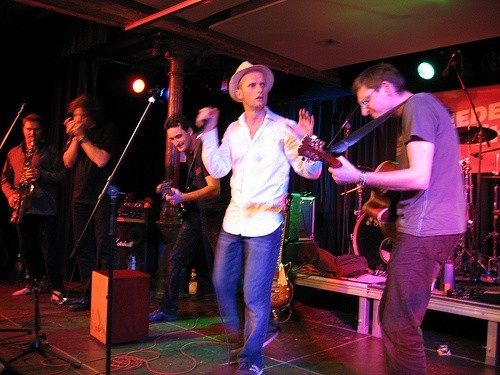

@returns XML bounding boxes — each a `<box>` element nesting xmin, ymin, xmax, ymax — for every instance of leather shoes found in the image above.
<box><xmin>68</xmin><ymin>298</ymin><xmax>90</xmax><ymax>311</ymax></box>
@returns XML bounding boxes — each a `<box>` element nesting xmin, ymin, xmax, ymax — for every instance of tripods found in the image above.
<box><xmin>453</xmin><ymin>136</ymin><xmax>500</xmax><ymax>281</ymax></box>
<box><xmin>4</xmin><ymin>271</ymin><xmax>83</xmax><ymax>370</ymax></box>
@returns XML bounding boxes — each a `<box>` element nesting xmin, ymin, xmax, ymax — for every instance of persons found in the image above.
<box><xmin>1</xmin><ymin>113</ymin><xmax>68</xmax><ymax>295</ymax></box>
<box><xmin>62</xmin><ymin>96</ymin><xmax>115</xmax><ymax>311</ymax></box>
<box><xmin>148</xmin><ymin>110</ymin><xmax>232</xmax><ymax>322</ymax></box>
<box><xmin>328</xmin><ymin>64</ymin><xmax>468</xmax><ymax>375</ymax></box>
<box><xmin>195</xmin><ymin>61</ymin><xmax>322</xmax><ymax>374</ymax></box>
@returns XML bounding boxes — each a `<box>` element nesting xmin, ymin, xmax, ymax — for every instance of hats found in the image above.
<box><xmin>229</xmin><ymin>62</ymin><xmax>274</xmax><ymax>101</ymax></box>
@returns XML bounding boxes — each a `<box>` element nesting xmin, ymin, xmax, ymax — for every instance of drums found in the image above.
<box><xmin>351</xmin><ymin>212</ymin><xmax>391</xmax><ymax>275</ymax></box>
<box><xmin>464</xmin><ymin>202</ymin><xmax>475</xmax><ymax>225</ymax></box>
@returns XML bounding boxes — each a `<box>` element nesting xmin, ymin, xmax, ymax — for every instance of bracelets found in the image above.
<box><xmin>359</xmin><ymin>168</ymin><xmax>368</xmax><ymax>188</ymax></box>
<box><xmin>79</xmin><ymin>136</ymin><xmax>89</xmax><ymax>144</ymax></box>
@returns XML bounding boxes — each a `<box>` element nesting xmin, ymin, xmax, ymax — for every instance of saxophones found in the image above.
<box><xmin>10</xmin><ymin>135</ymin><xmax>36</xmax><ymax>225</ymax></box>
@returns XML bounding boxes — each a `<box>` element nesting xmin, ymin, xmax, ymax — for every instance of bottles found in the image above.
<box><xmin>189</xmin><ymin>268</ymin><xmax>199</xmax><ymax>297</ymax></box>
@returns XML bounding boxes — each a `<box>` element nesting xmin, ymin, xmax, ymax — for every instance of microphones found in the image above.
<box><xmin>443</xmin><ymin>52</ymin><xmax>457</xmax><ymax>77</ymax></box>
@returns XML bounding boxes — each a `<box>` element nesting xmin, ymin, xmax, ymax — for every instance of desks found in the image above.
<box><xmin>116</xmin><ymin>217</ymin><xmax>183</xmax><ymax>226</ymax></box>
<box><xmin>292</xmin><ymin>274</ymin><xmax>500</xmax><ymax>366</ymax></box>
<box><xmin>89</xmin><ymin>269</ymin><xmax>150</xmax><ymax>345</ymax></box>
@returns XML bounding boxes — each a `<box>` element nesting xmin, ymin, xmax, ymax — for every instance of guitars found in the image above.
<box><xmin>294</xmin><ymin>132</ymin><xmax>408</xmax><ymax>242</ymax></box>
<box><xmin>267</xmin><ymin>194</ymin><xmax>294</xmax><ymax>310</ymax></box>
<box><xmin>160</xmin><ymin>179</ymin><xmax>187</xmax><ymax>222</ymax></box>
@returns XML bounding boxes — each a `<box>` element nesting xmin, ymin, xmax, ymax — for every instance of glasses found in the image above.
<box><xmin>359</xmin><ymin>83</ymin><xmax>381</xmax><ymax>108</ymax></box>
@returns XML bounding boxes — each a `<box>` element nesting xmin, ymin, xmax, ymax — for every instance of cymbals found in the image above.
<box><xmin>453</xmin><ymin>126</ymin><xmax>497</xmax><ymax>144</ymax></box>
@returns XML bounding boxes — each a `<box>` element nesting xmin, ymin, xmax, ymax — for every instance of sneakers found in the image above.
<box><xmin>236</xmin><ymin>361</ymin><xmax>262</xmax><ymax>375</ymax></box>
<box><xmin>262</xmin><ymin>329</ymin><xmax>279</xmax><ymax>348</ymax></box>
<box><xmin>148</xmin><ymin>309</ymin><xmax>176</xmax><ymax>322</ymax></box>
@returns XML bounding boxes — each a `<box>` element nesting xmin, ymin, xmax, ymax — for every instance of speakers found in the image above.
<box><xmin>90</xmin><ymin>269</ymin><xmax>151</xmax><ymax>344</ymax></box>
<box><xmin>284</xmin><ymin>192</ymin><xmax>319</xmax><ymax>268</ymax></box>
<box><xmin>114</xmin><ymin>211</ymin><xmax>150</xmax><ymax>272</ymax></box>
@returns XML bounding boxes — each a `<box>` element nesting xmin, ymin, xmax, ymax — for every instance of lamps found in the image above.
<box><xmin>416</xmin><ymin>49</ymin><xmax>443</xmax><ymax>80</ymax></box>
